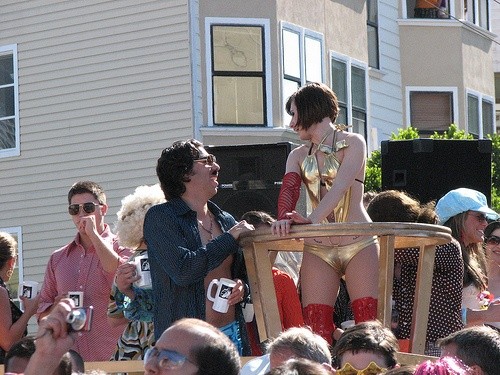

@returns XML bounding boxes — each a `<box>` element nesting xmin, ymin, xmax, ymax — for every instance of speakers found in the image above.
<box><xmin>382</xmin><ymin>138</ymin><xmax>492</xmax><ymax>211</ymax></box>
<box><xmin>203</xmin><ymin>140</ymin><xmax>292</xmax><ymax>223</ymax></box>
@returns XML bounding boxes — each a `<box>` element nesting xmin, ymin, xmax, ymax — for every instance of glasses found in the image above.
<box><xmin>192</xmin><ymin>155</ymin><xmax>216</xmax><ymax>167</ymax></box>
<box><xmin>144</xmin><ymin>346</ymin><xmax>197</xmax><ymax>370</ymax></box>
<box><xmin>335</xmin><ymin>361</ymin><xmax>392</xmax><ymax>375</ymax></box>
<box><xmin>485</xmin><ymin>236</ymin><xmax>500</xmax><ymax>245</ymax></box>
<box><xmin>68</xmin><ymin>202</ymin><xmax>103</xmax><ymax>214</ymax></box>
<box><xmin>467</xmin><ymin>213</ymin><xmax>487</xmax><ymax>222</ymax></box>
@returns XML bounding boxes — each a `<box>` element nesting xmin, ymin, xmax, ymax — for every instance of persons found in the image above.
<box><xmin>0</xmin><ymin>81</ymin><xmax>500</xmax><ymax>375</ymax></box>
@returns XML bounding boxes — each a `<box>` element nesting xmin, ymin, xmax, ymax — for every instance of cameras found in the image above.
<box><xmin>65</xmin><ymin>306</ymin><xmax>94</xmax><ymax>334</ymax></box>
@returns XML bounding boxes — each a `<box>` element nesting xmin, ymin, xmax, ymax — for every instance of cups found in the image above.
<box><xmin>21</xmin><ymin>281</ymin><xmax>37</xmax><ymax>309</ymax></box>
<box><xmin>68</xmin><ymin>291</ymin><xmax>83</xmax><ymax>308</ymax></box>
<box><xmin>207</xmin><ymin>277</ymin><xmax>237</xmax><ymax>313</ymax></box>
<box><xmin>130</xmin><ymin>256</ymin><xmax>153</xmax><ymax>289</ymax></box>
<box><xmin>341</xmin><ymin>320</ymin><xmax>356</xmax><ymax>332</ymax></box>
<box><xmin>241</xmin><ymin>295</ymin><xmax>254</xmax><ymax>323</ymax></box>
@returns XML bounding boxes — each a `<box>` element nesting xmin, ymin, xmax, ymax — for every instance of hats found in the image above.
<box><xmin>433</xmin><ymin>188</ymin><xmax>499</xmax><ymax>225</ymax></box>
